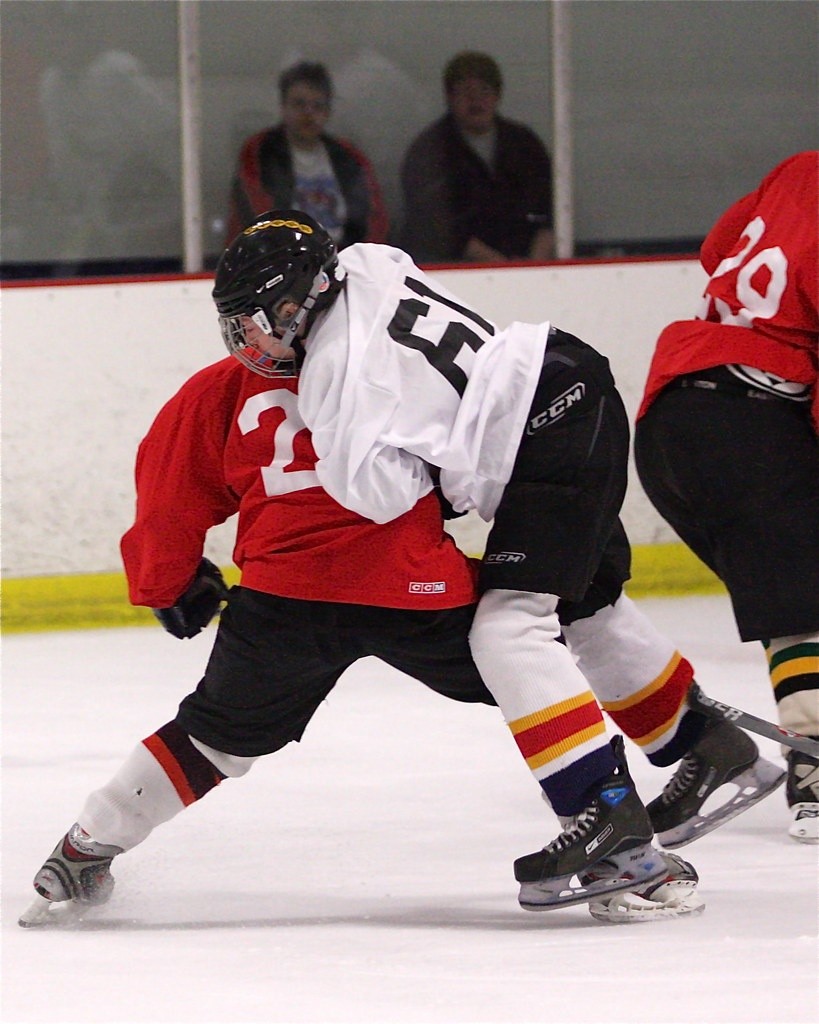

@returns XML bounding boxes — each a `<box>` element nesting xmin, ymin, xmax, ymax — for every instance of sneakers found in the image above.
<box><xmin>646</xmin><ymin>680</ymin><xmax>788</xmax><ymax>849</ymax></box>
<box><xmin>512</xmin><ymin>735</ymin><xmax>669</xmax><ymax>913</ymax></box>
<box><xmin>785</xmin><ymin>734</ymin><xmax>819</xmax><ymax>839</ymax></box>
<box><xmin>582</xmin><ymin>849</ymin><xmax>707</xmax><ymax>923</ymax></box>
<box><xmin>18</xmin><ymin>822</ymin><xmax>126</xmax><ymax>926</ymax></box>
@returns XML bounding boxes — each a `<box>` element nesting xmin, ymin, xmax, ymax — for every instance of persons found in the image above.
<box><xmin>221</xmin><ymin>59</ymin><xmax>389</xmax><ymax>254</ymax></box>
<box><xmin>212</xmin><ymin>208</ymin><xmax>786</xmax><ymax>884</ymax></box>
<box><xmin>11</xmin><ymin>337</ymin><xmax>698</xmax><ymax>903</ymax></box>
<box><xmin>397</xmin><ymin>51</ymin><xmax>555</xmax><ymax>269</ymax></box>
<box><xmin>635</xmin><ymin>148</ymin><xmax>819</xmax><ymax>845</ymax></box>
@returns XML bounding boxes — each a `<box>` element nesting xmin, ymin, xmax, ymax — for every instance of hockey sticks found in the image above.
<box><xmin>556</xmin><ymin>631</ymin><xmax>819</xmax><ymax>760</ymax></box>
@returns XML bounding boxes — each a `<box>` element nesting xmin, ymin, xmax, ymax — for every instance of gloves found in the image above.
<box><xmin>424</xmin><ymin>464</ymin><xmax>471</xmax><ymax>520</ymax></box>
<box><xmin>153</xmin><ymin>558</ymin><xmax>225</xmax><ymax>639</ymax></box>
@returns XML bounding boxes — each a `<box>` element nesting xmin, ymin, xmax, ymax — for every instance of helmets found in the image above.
<box><xmin>212</xmin><ymin>209</ymin><xmax>349</xmax><ymax>379</ymax></box>
